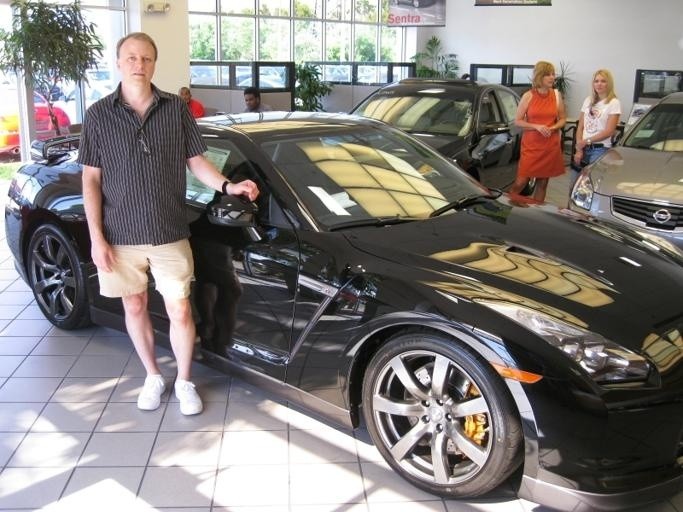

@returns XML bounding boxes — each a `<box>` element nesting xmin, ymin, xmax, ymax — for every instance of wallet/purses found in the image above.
<box><xmin>571</xmin><ymin>159</ymin><xmax>589</xmax><ymax>173</ymax></box>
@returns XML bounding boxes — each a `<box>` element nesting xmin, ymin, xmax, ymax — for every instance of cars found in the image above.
<box><xmin>4</xmin><ymin>110</ymin><xmax>683</xmax><ymax>511</ymax></box>
<box><xmin>347</xmin><ymin>79</ymin><xmax>538</xmax><ymax>197</ymax></box>
<box><xmin>212</xmin><ymin>66</ymin><xmax>282</xmax><ymax>85</ymax></box>
<box><xmin>63</xmin><ymin>69</ymin><xmax>111</xmax><ymax>100</ymax></box>
<box><xmin>192</xmin><ymin>66</ymin><xmax>216</xmax><ymax>84</ymax></box>
<box><xmin>568</xmin><ymin>92</ymin><xmax>683</xmax><ymax>251</ymax></box>
<box><xmin>53</xmin><ymin>80</ymin><xmax>114</xmax><ymax>124</ymax></box>
<box><xmin>0</xmin><ymin>83</ymin><xmax>76</xmax><ymax>154</ymax></box>
<box><xmin>33</xmin><ymin>79</ymin><xmax>60</xmax><ymax>101</ymax></box>
<box><xmin>327</xmin><ymin>66</ymin><xmax>363</xmax><ymax>81</ymax></box>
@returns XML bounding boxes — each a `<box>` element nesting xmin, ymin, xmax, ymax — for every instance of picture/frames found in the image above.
<box><xmin>473</xmin><ymin>0</ymin><xmax>552</xmax><ymax>7</ymax></box>
<box><xmin>385</xmin><ymin>0</ymin><xmax>445</xmax><ymax>28</ymax></box>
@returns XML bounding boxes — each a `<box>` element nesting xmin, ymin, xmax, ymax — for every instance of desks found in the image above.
<box><xmin>559</xmin><ymin>118</ymin><xmax>626</xmax><ymax>166</ymax></box>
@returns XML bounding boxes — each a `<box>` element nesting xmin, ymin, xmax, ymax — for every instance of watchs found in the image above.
<box><xmin>222</xmin><ymin>178</ymin><xmax>231</xmax><ymax>197</ymax></box>
<box><xmin>587</xmin><ymin>137</ymin><xmax>591</xmax><ymax>145</ymax></box>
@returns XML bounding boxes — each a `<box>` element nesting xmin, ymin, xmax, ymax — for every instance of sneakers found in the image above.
<box><xmin>175</xmin><ymin>379</ymin><xmax>203</xmax><ymax>415</ymax></box>
<box><xmin>138</xmin><ymin>374</ymin><xmax>166</xmax><ymax>411</ymax></box>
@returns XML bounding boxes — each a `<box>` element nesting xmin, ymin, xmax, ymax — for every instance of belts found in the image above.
<box><xmin>586</xmin><ymin>143</ymin><xmax>603</xmax><ymax>148</ymax></box>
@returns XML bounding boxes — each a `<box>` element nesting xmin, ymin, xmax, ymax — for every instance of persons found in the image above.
<box><xmin>510</xmin><ymin>62</ymin><xmax>567</xmax><ymax>204</ymax></box>
<box><xmin>179</xmin><ymin>87</ymin><xmax>204</xmax><ymax>119</ymax></box>
<box><xmin>244</xmin><ymin>86</ymin><xmax>272</xmax><ymax>113</ymax></box>
<box><xmin>573</xmin><ymin>69</ymin><xmax>622</xmax><ymax>167</ymax></box>
<box><xmin>77</xmin><ymin>31</ymin><xmax>260</xmax><ymax>416</ymax></box>
